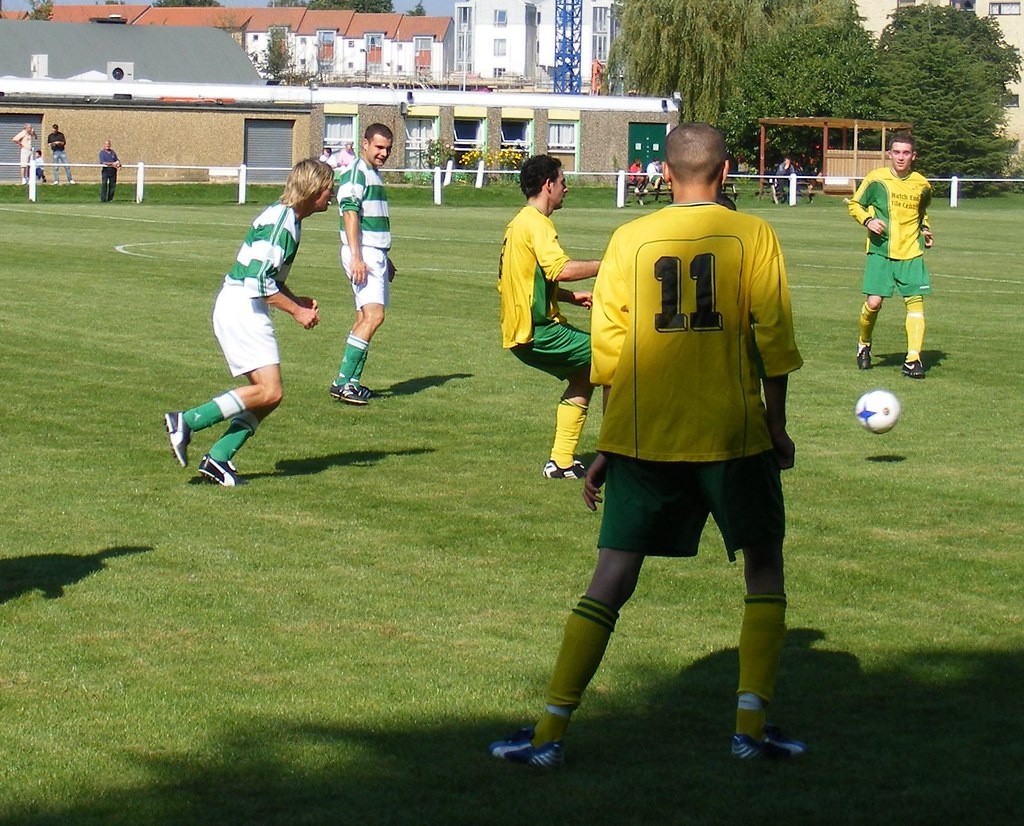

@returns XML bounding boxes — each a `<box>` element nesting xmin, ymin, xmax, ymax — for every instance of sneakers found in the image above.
<box><xmin>542</xmin><ymin>460</ymin><xmax>588</xmax><ymax>479</ymax></box>
<box><xmin>857</xmin><ymin>343</ymin><xmax>871</xmax><ymax>369</ymax></box>
<box><xmin>356</xmin><ymin>383</ymin><xmax>376</xmax><ymax>399</ymax></box>
<box><xmin>164</xmin><ymin>411</ymin><xmax>192</xmax><ymax>468</ymax></box>
<box><xmin>901</xmin><ymin>359</ymin><xmax>926</xmax><ymax>378</ymax></box>
<box><xmin>732</xmin><ymin>725</ymin><xmax>809</xmax><ymax>759</ymax></box>
<box><xmin>330</xmin><ymin>380</ymin><xmax>369</xmax><ymax>404</ymax></box>
<box><xmin>488</xmin><ymin>728</ymin><xmax>566</xmax><ymax>771</ymax></box>
<box><xmin>197</xmin><ymin>454</ymin><xmax>247</xmax><ymax>486</ymax></box>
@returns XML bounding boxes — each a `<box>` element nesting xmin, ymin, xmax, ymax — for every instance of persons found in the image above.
<box><xmin>98</xmin><ymin>140</ymin><xmax>121</xmax><ymax>202</ymax></box>
<box><xmin>35</xmin><ymin>150</ymin><xmax>47</xmax><ymax>183</ymax></box>
<box><xmin>498</xmin><ymin>155</ymin><xmax>602</xmax><ymax>481</ymax></box>
<box><xmin>338</xmin><ymin>140</ymin><xmax>356</xmax><ymax>168</ymax></box>
<box><xmin>330</xmin><ymin>123</ymin><xmax>398</xmax><ymax>405</ymax></box>
<box><xmin>647</xmin><ymin>158</ymin><xmax>663</xmax><ymax>189</ymax></box>
<box><xmin>166</xmin><ymin>160</ymin><xmax>335</xmax><ymax>487</ymax></box>
<box><xmin>488</xmin><ymin>122</ymin><xmax>808</xmax><ymax>769</ymax></box>
<box><xmin>848</xmin><ymin>136</ymin><xmax>934</xmax><ymax>378</ymax></box>
<box><xmin>630</xmin><ymin>158</ymin><xmax>649</xmax><ymax>193</ymax></box>
<box><xmin>47</xmin><ymin>124</ymin><xmax>76</xmax><ymax>185</ymax></box>
<box><xmin>320</xmin><ymin>148</ymin><xmax>337</xmax><ymax>168</ymax></box>
<box><xmin>775</xmin><ymin>156</ymin><xmax>794</xmax><ymax>191</ymax></box>
<box><xmin>13</xmin><ymin>123</ymin><xmax>38</xmax><ymax>185</ymax></box>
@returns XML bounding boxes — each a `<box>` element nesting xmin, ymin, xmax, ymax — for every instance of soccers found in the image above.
<box><xmin>853</xmin><ymin>390</ymin><xmax>902</xmax><ymax>434</ymax></box>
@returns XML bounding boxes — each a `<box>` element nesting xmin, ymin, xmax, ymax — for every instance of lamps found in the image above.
<box><xmin>400</xmin><ymin>102</ymin><xmax>407</xmax><ymax>121</ymax></box>
<box><xmin>310</xmin><ymin>79</ymin><xmax>319</xmax><ymax>105</ymax></box>
<box><xmin>662</xmin><ymin>100</ymin><xmax>667</xmax><ymax>108</ymax></box>
<box><xmin>407</xmin><ymin>92</ymin><xmax>412</xmax><ymax>100</ymax></box>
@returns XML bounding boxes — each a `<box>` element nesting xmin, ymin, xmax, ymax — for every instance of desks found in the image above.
<box><xmin>623</xmin><ymin>182</ymin><xmax>739</xmax><ymax>203</ymax></box>
<box><xmin>758</xmin><ymin>181</ymin><xmax>817</xmax><ymax>203</ymax></box>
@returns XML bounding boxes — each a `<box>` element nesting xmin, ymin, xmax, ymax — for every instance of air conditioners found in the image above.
<box><xmin>107</xmin><ymin>61</ymin><xmax>136</xmax><ymax>82</ymax></box>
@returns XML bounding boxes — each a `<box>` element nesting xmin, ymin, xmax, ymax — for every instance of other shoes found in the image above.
<box><xmin>68</xmin><ymin>179</ymin><xmax>76</xmax><ymax>184</ymax></box>
<box><xmin>54</xmin><ymin>181</ymin><xmax>59</xmax><ymax>185</ymax></box>
<box><xmin>634</xmin><ymin>188</ymin><xmax>640</xmax><ymax>193</ymax></box>
<box><xmin>642</xmin><ymin>190</ymin><xmax>649</xmax><ymax>194</ymax></box>
<box><xmin>22</xmin><ymin>178</ymin><xmax>28</xmax><ymax>184</ymax></box>
<box><xmin>655</xmin><ymin>189</ymin><xmax>661</xmax><ymax>193</ymax></box>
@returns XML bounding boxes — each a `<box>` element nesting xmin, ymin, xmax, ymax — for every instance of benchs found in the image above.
<box><xmin>624</xmin><ymin>189</ymin><xmax>820</xmax><ymax>198</ymax></box>
